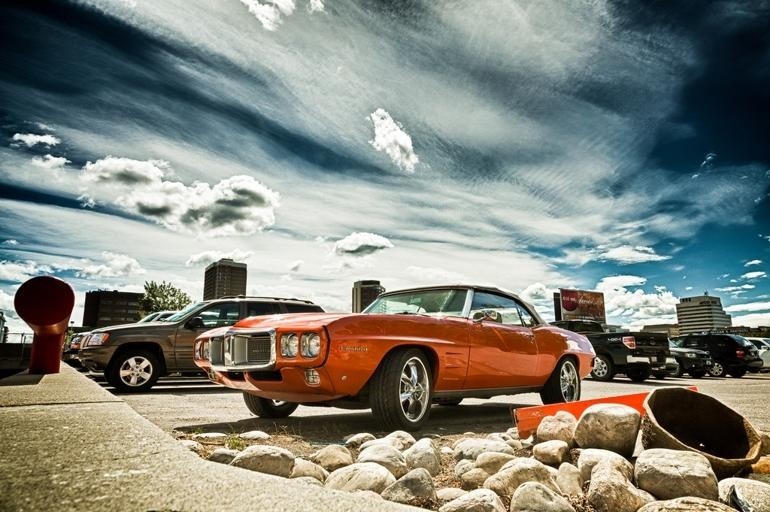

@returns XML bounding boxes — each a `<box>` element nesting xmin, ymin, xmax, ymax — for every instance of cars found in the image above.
<box><xmin>664</xmin><ymin>333</ymin><xmax>770</xmax><ymax>377</ymax></box>
<box><xmin>60</xmin><ymin>311</ymin><xmax>240</xmax><ymax>388</ymax></box>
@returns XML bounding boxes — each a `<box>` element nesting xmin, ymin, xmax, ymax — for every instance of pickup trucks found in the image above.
<box><xmin>544</xmin><ymin>320</ymin><xmax>671</xmax><ymax>381</ymax></box>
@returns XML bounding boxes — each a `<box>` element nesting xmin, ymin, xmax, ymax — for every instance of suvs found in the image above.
<box><xmin>78</xmin><ymin>294</ymin><xmax>326</xmax><ymax>393</ymax></box>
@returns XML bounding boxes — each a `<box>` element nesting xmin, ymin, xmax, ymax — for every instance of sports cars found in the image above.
<box><xmin>192</xmin><ymin>286</ymin><xmax>598</xmax><ymax>432</ymax></box>
